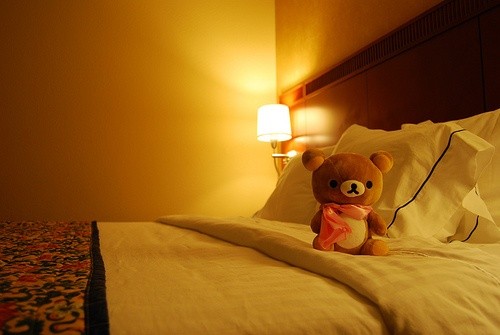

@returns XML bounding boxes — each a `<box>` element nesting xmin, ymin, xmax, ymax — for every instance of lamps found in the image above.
<box><xmin>257</xmin><ymin>104</ymin><xmax>293</xmax><ymax>177</ymax></box>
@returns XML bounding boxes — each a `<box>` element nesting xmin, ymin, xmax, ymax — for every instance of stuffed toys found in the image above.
<box><xmin>301</xmin><ymin>150</ymin><xmax>394</xmax><ymax>256</ymax></box>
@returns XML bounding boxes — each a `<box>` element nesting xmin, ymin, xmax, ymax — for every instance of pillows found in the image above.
<box><xmin>252</xmin><ymin>144</ymin><xmax>336</xmax><ymax>226</ymax></box>
<box><xmin>330</xmin><ymin>123</ymin><xmax>498</xmax><ymax>243</ymax></box>
<box><xmin>401</xmin><ymin>108</ymin><xmax>500</xmax><ymax>244</ymax></box>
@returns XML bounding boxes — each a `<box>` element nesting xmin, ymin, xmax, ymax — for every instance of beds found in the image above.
<box><xmin>0</xmin><ymin>214</ymin><xmax>500</xmax><ymax>335</ymax></box>
